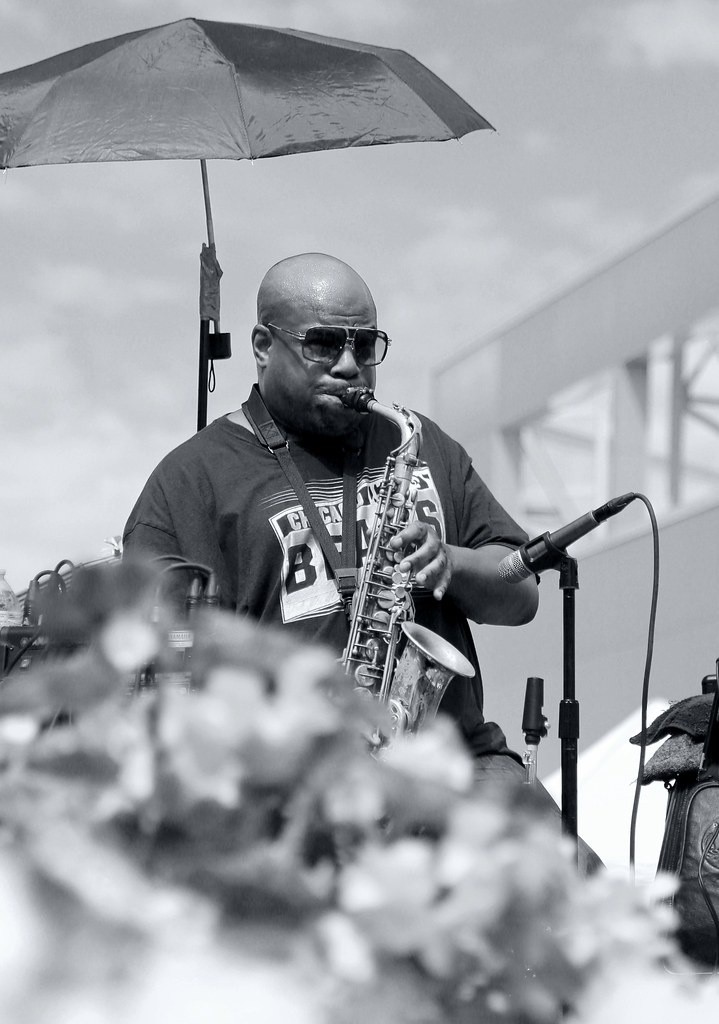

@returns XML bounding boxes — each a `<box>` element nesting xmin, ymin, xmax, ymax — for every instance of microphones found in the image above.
<box><xmin>497</xmin><ymin>493</ymin><xmax>635</xmax><ymax>585</ymax></box>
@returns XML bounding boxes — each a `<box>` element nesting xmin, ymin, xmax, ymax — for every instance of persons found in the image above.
<box><xmin>121</xmin><ymin>252</ymin><xmax>609</xmax><ymax>869</ymax></box>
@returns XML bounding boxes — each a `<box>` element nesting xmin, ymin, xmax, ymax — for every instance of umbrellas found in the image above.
<box><xmin>0</xmin><ymin>18</ymin><xmax>496</xmax><ymax>432</ymax></box>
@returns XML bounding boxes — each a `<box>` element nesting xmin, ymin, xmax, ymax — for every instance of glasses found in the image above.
<box><xmin>261</xmin><ymin>320</ymin><xmax>390</xmax><ymax>367</ymax></box>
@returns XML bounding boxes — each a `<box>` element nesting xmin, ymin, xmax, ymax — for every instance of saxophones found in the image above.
<box><xmin>337</xmin><ymin>384</ymin><xmax>479</xmax><ymax>760</ymax></box>
